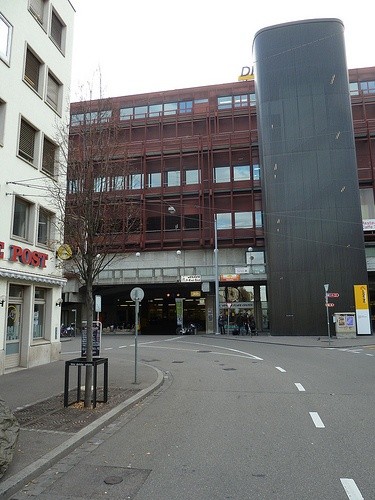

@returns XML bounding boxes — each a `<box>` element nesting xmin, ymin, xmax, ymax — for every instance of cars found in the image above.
<box><xmin>175</xmin><ymin>324</ymin><xmax>196</xmax><ymax>335</ymax></box>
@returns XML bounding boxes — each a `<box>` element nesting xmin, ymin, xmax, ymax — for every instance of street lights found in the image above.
<box><xmin>72</xmin><ymin>309</ymin><xmax>76</xmax><ymax>337</ymax></box>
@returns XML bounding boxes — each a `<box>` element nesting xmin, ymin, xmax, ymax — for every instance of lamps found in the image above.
<box><xmin>248</xmin><ymin>247</ymin><xmax>254</xmax><ymax>273</ymax></box>
<box><xmin>56</xmin><ymin>298</ymin><xmax>64</xmax><ymax>307</ymax></box>
<box><xmin>96</xmin><ymin>253</ymin><xmax>102</xmax><ymax>268</ymax></box>
<box><xmin>135</xmin><ymin>252</ymin><xmax>141</xmax><ymax>271</ymax></box>
<box><xmin>176</xmin><ymin>250</ymin><xmax>181</xmax><ymax>269</ymax></box>
<box><xmin>168</xmin><ymin>205</ymin><xmax>175</xmax><ymax>212</ymax></box>
<box><xmin>0</xmin><ymin>294</ymin><xmax>6</xmax><ymax>307</ymax></box>
